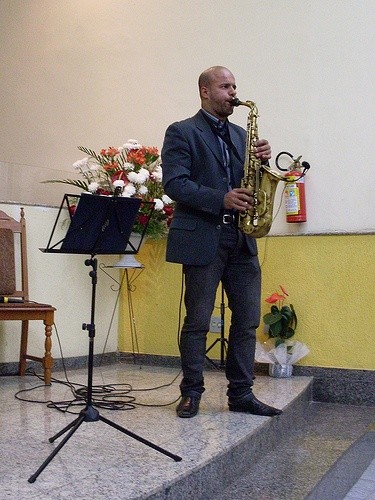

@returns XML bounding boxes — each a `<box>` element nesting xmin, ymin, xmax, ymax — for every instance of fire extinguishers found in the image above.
<box><xmin>275</xmin><ymin>150</ymin><xmax>311</xmax><ymax>222</ymax></box>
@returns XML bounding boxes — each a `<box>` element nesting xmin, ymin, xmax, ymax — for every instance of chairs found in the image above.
<box><xmin>0</xmin><ymin>206</ymin><xmax>58</xmax><ymax>386</ymax></box>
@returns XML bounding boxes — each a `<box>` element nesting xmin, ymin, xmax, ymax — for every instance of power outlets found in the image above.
<box><xmin>208</xmin><ymin>315</ymin><xmax>227</xmax><ymax>333</ymax></box>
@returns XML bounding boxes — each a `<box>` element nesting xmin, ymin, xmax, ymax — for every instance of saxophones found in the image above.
<box><xmin>230</xmin><ymin>96</ymin><xmax>284</xmax><ymax>238</ymax></box>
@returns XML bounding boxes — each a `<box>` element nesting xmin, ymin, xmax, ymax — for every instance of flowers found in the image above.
<box><xmin>254</xmin><ymin>285</ymin><xmax>310</xmax><ymax>366</ymax></box>
<box><xmin>38</xmin><ymin>139</ymin><xmax>175</xmax><ymax>242</ymax></box>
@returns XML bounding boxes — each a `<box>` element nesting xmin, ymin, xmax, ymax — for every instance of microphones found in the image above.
<box><xmin>291</xmin><ymin>161</ymin><xmax>310</xmax><ymax>181</ymax></box>
<box><xmin>0</xmin><ymin>296</ymin><xmax>25</xmax><ymax>303</ymax></box>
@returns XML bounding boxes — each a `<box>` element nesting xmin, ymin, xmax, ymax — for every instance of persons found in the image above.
<box><xmin>160</xmin><ymin>65</ymin><xmax>283</xmax><ymax>418</ymax></box>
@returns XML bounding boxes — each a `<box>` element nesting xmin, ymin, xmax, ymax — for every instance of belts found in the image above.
<box><xmin>222</xmin><ymin>214</ymin><xmax>236</xmax><ymax>224</ymax></box>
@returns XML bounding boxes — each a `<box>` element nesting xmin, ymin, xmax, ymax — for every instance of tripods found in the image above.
<box><xmin>204</xmin><ymin>282</ymin><xmax>256</xmax><ymax>381</ymax></box>
<box><xmin>27</xmin><ymin>192</ymin><xmax>181</xmax><ymax>484</ymax></box>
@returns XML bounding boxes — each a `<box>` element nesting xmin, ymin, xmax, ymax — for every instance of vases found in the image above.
<box><xmin>268</xmin><ymin>362</ymin><xmax>293</xmax><ymax>378</ymax></box>
<box><xmin>112</xmin><ymin>231</ymin><xmax>147</xmax><ymax>269</ymax></box>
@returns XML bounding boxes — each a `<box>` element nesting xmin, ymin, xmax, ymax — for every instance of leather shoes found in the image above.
<box><xmin>176</xmin><ymin>399</ymin><xmax>199</xmax><ymax>418</ymax></box>
<box><xmin>228</xmin><ymin>394</ymin><xmax>283</xmax><ymax>416</ymax></box>
